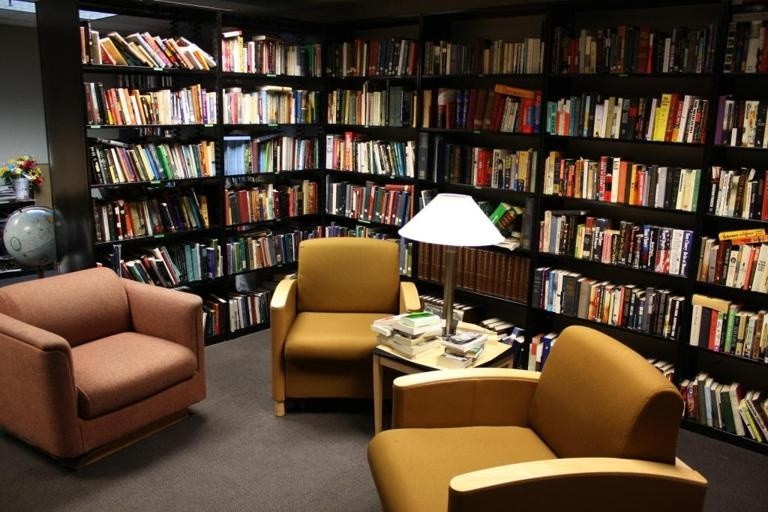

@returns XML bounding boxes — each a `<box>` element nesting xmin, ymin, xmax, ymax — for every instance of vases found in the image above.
<box><xmin>12</xmin><ymin>176</ymin><xmax>30</xmax><ymax>200</ymax></box>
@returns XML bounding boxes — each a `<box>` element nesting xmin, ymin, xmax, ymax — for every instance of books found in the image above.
<box><xmin>79</xmin><ymin>11</ymin><xmax>767</xmax><ymax>445</ymax></box>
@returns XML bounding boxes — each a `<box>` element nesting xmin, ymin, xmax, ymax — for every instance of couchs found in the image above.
<box><xmin>3</xmin><ymin>266</ymin><xmax>208</xmax><ymax>470</ymax></box>
<box><xmin>269</xmin><ymin>237</ymin><xmax>421</xmax><ymax>418</ymax></box>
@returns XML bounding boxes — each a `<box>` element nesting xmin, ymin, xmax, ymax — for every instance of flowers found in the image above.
<box><xmin>0</xmin><ymin>150</ymin><xmax>45</xmax><ymax>186</ymax></box>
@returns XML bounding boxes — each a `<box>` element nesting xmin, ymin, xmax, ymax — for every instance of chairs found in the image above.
<box><xmin>367</xmin><ymin>321</ymin><xmax>709</xmax><ymax>510</ymax></box>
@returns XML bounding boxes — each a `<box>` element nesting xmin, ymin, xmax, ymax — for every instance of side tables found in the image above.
<box><xmin>370</xmin><ymin>322</ymin><xmax>514</xmax><ymax>444</ymax></box>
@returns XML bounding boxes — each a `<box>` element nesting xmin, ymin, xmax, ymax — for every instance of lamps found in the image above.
<box><xmin>395</xmin><ymin>195</ymin><xmax>512</xmax><ymax>346</ymax></box>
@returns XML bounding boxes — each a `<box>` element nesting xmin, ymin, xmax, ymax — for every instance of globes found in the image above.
<box><xmin>2</xmin><ymin>205</ymin><xmax>57</xmax><ymax>278</ymax></box>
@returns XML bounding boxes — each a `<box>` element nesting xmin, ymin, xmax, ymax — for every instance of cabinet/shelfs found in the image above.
<box><xmin>319</xmin><ymin>2</ymin><xmax>766</xmax><ymax>454</ymax></box>
<box><xmin>35</xmin><ymin>2</ymin><xmax>325</xmax><ymax>347</ymax></box>
